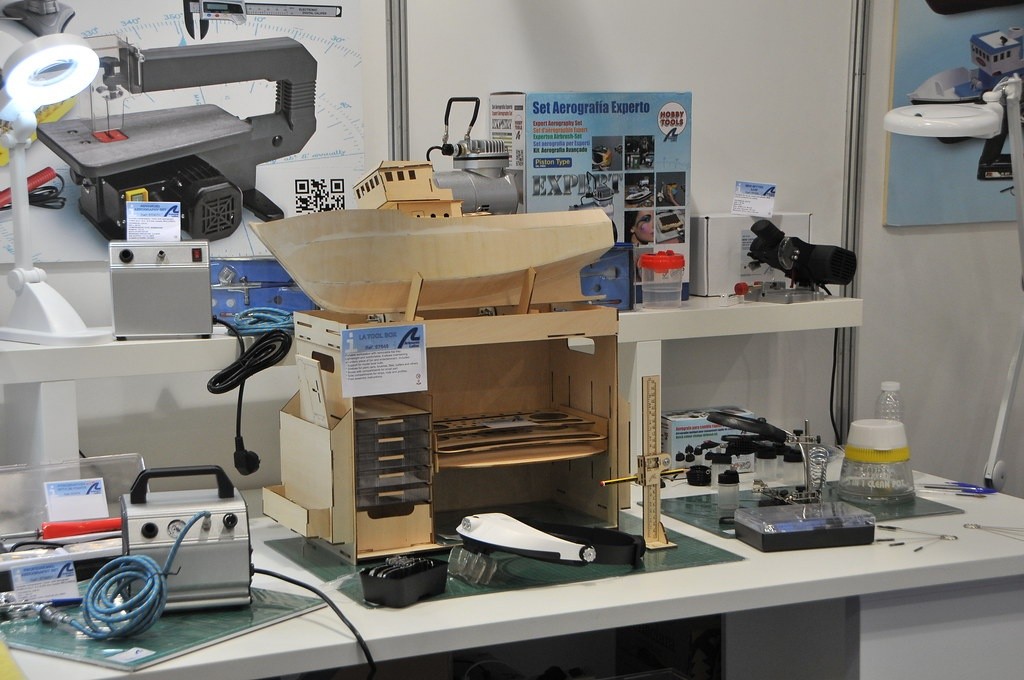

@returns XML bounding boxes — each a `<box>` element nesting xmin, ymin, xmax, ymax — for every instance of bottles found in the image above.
<box><xmin>752</xmin><ymin>438</ymin><xmax>803</xmax><ymax>486</ymax></box>
<box><xmin>875</xmin><ymin>381</ymin><xmax>901</xmax><ymax>422</ymax></box>
<box><xmin>718</xmin><ymin>468</ymin><xmax>741</xmax><ymax>526</ymax></box>
<box><xmin>838</xmin><ymin>419</ymin><xmax>915</xmax><ymax>507</ymax></box>
<box><xmin>711</xmin><ymin>455</ymin><xmax>731</xmax><ymax>490</ymax></box>
<box><xmin>675</xmin><ymin>441</ymin><xmax>713</xmax><ymax>469</ymax></box>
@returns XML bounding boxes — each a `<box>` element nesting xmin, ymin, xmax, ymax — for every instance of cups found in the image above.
<box><xmin>639</xmin><ymin>251</ymin><xmax>685</xmax><ymax>309</ymax></box>
<box><xmin>686</xmin><ymin>465</ymin><xmax>711</xmax><ymax>487</ymax></box>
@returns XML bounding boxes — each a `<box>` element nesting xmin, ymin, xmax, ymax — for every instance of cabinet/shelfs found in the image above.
<box><xmin>0</xmin><ymin>288</ymin><xmax>1023</xmax><ymax>679</ymax></box>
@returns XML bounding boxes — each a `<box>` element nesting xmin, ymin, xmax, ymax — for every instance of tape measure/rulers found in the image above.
<box><xmin>188</xmin><ymin>2</ymin><xmax>337</xmax><ymax>18</ymax></box>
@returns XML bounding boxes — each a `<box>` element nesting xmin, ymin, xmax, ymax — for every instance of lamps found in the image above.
<box><xmin>2</xmin><ymin>33</ymin><xmax>102</xmax><ymax>293</ymax></box>
<box><xmin>881</xmin><ymin>73</ymin><xmax>1023</xmax><ymax>495</ymax></box>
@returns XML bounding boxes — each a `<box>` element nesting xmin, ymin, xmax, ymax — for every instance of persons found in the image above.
<box><xmin>624</xmin><ymin>211</ymin><xmax>655</xmax><ymax>245</ymax></box>
